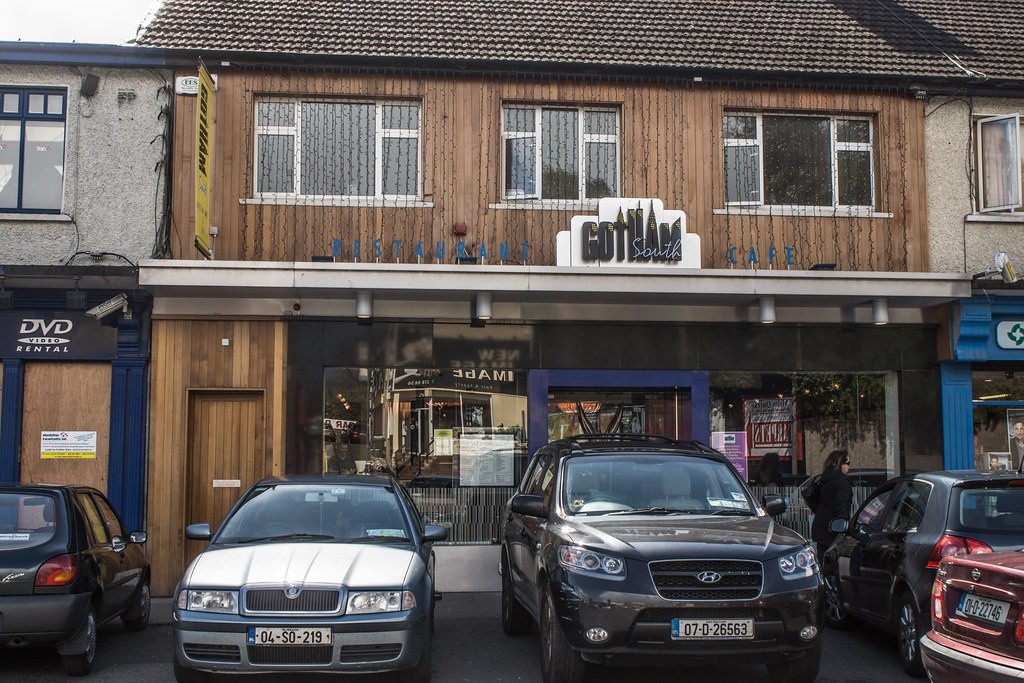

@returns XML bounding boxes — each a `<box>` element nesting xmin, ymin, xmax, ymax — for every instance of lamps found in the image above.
<box><xmin>475</xmin><ymin>292</ymin><xmax>493</xmax><ymax>320</ymax></box>
<box><xmin>811</xmin><ymin>263</ymin><xmax>836</xmax><ymax>271</ymax></box>
<box><xmin>312</xmin><ymin>256</ymin><xmax>333</xmax><ymax>262</ymax></box>
<box><xmin>455</xmin><ymin>256</ymin><xmax>477</xmax><ymax>264</ymax></box>
<box><xmin>871</xmin><ymin>297</ymin><xmax>889</xmax><ymax>326</ymax></box>
<box><xmin>357</xmin><ymin>291</ymin><xmax>373</xmax><ymax>319</ymax></box>
<box><xmin>760</xmin><ymin>297</ymin><xmax>776</xmax><ymax>325</ymax></box>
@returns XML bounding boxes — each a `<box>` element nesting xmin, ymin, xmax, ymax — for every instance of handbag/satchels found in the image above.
<box><xmin>801</xmin><ymin>483</ymin><xmax>820</xmax><ymax>514</ymax></box>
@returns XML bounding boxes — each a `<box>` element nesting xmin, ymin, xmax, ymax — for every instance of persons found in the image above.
<box><xmin>341</xmin><ymin>427</ymin><xmax>360</xmax><ymax>460</ymax></box>
<box><xmin>329</xmin><ymin>444</ymin><xmax>357</xmax><ymax>474</ymax></box>
<box><xmin>370</xmin><ymin>458</ymin><xmax>395</xmax><ymax>478</ymax></box>
<box><xmin>1010</xmin><ymin>417</ymin><xmax>1024</xmax><ymax>469</ymax></box>
<box><xmin>990</xmin><ymin>455</ymin><xmax>1006</xmax><ymax>470</ymax></box>
<box><xmin>754</xmin><ymin>453</ymin><xmax>799</xmax><ymax>532</ymax></box>
<box><xmin>812</xmin><ymin>450</ymin><xmax>853</xmax><ymax>563</ymax></box>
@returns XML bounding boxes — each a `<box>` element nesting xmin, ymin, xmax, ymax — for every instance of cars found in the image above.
<box><xmin>408</xmin><ymin>447</ymin><xmax>530</xmax><ymax>544</ymax></box>
<box><xmin>918</xmin><ymin>550</ymin><xmax>1024</xmax><ymax>683</ymax></box>
<box><xmin>324</xmin><ymin>422</ymin><xmax>336</xmax><ymax>442</ymax></box>
<box><xmin>170</xmin><ymin>473</ymin><xmax>449</xmax><ymax>682</ymax></box>
<box><xmin>0</xmin><ymin>481</ymin><xmax>151</xmax><ymax>674</ymax></box>
<box><xmin>770</xmin><ymin>469</ymin><xmax>931</xmax><ymax>563</ymax></box>
<box><xmin>820</xmin><ymin>468</ymin><xmax>1024</xmax><ymax>676</ymax></box>
<box><xmin>344</xmin><ymin>423</ymin><xmax>367</xmax><ymax>444</ymax></box>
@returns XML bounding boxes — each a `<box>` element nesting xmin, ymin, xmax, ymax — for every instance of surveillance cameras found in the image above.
<box><xmin>84</xmin><ymin>293</ymin><xmax>128</xmax><ymax>320</ymax></box>
<box><xmin>995</xmin><ymin>253</ymin><xmax>1017</xmax><ymax>283</ymax></box>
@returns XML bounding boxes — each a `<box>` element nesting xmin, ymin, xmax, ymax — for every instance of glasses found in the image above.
<box><xmin>843</xmin><ymin>461</ymin><xmax>850</xmax><ymax>465</ymax></box>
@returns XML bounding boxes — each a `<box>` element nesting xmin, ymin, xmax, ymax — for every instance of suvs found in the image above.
<box><xmin>497</xmin><ymin>431</ymin><xmax>825</xmax><ymax>683</ymax></box>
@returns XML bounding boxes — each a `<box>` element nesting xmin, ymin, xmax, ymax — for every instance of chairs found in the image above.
<box><xmin>571</xmin><ymin>467</ymin><xmax>603</xmax><ymax>503</ymax></box>
<box><xmin>352</xmin><ymin>501</ymin><xmax>393</xmax><ymax>534</ymax></box>
<box><xmin>262</xmin><ymin>499</ymin><xmax>303</xmax><ymax>530</ymax></box>
<box><xmin>33</xmin><ymin>502</ymin><xmax>54</xmax><ymax>534</ymax></box>
<box><xmin>650</xmin><ymin>475</ymin><xmax>706</xmax><ymax>511</ymax></box>
<box><xmin>993</xmin><ymin>494</ymin><xmax>1024</xmax><ymax>528</ymax></box>
